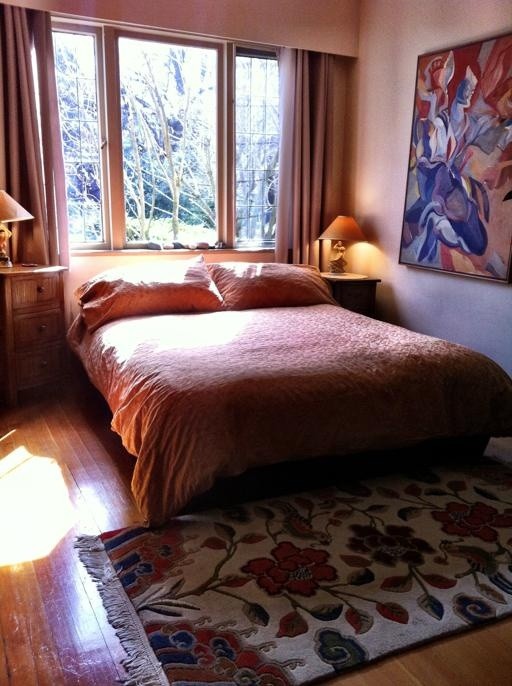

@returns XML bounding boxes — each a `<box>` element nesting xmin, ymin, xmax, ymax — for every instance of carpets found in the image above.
<box><xmin>74</xmin><ymin>456</ymin><xmax>512</xmax><ymax>686</ymax></box>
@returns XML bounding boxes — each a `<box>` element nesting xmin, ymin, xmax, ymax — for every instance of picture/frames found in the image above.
<box><xmin>398</xmin><ymin>31</ymin><xmax>512</xmax><ymax>284</ymax></box>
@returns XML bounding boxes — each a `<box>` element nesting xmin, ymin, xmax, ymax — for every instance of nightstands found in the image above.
<box><xmin>0</xmin><ymin>263</ymin><xmax>70</xmax><ymax>408</ymax></box>
<box><xmin>321</xmin><ymin>275</ymin><xmax>381</xmax><ymax>319</ymax></box>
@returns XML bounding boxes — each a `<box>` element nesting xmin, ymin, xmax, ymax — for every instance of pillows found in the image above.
<box><xmin>73</xmin><ymin>253</ymin><xmax>341</xmax><ymax>335</ymax></box>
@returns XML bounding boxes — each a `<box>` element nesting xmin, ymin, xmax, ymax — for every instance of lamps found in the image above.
<box><xmin>0</xmin><ymin>189</ymin><xmax>35</xmax><ymax>268</ymax></box>
<box><xmin>317</xmin><ymin>216</ymin><xmax>368</xmax><ymax>274</ymax></box>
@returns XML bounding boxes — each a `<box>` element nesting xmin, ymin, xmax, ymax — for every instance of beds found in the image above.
<box><xmin>66</xmin><ymin>304</ymin><xmax>512</xmax><ymax>528</ymax></box>
